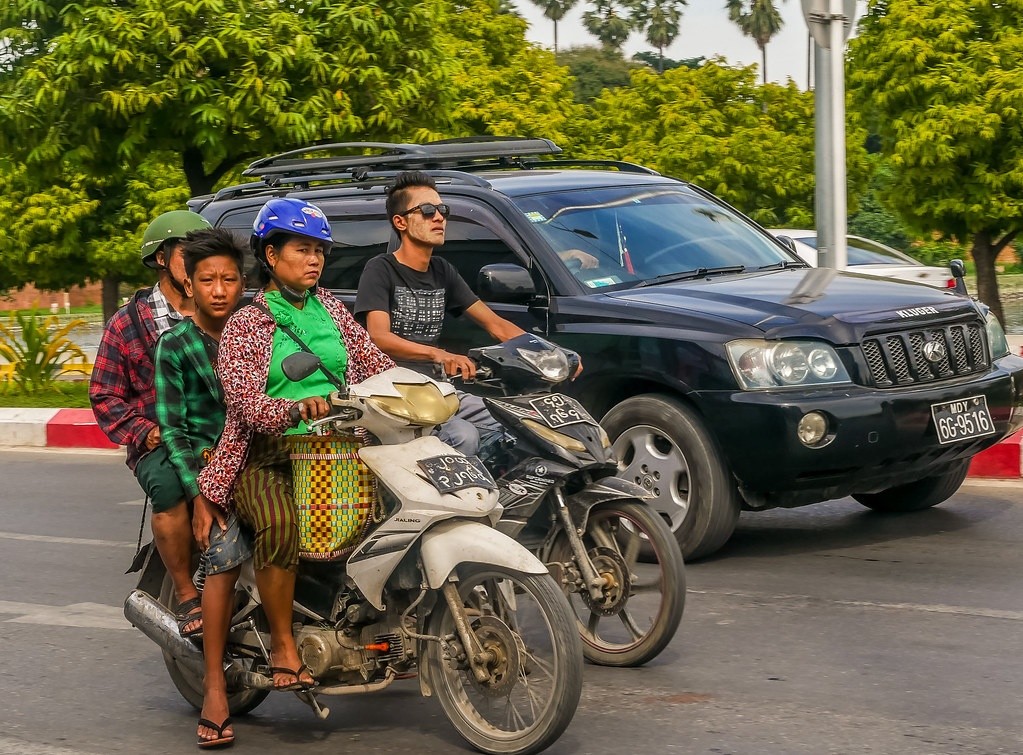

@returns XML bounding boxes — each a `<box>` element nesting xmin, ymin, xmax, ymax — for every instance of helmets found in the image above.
<box><xmin>141</xmin><ymin>211</ymin><xmax>213</xmax><ymax>268</ymax></box>
<box><xmin>253</xmin><ymin>198</ymin><xmax>332</xmax><ymax>242</ymax></box>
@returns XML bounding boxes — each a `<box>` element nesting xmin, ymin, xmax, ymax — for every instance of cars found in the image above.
<box><xmin>645</xmin><ymin>228</ymin><xmax>956</xmax><ymax>294</ymax></box>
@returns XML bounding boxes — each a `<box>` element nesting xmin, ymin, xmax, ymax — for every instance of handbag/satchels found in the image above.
<box><xmin>288</xmin><ymin>433</ymin><xmax>384</xmax><ymax>561</ymax></box>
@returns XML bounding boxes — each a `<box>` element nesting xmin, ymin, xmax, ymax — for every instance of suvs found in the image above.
<box><xmin>183</xmin><ymin>136</ymin><xmax>1023</xmax><ymax>564</ymax></box>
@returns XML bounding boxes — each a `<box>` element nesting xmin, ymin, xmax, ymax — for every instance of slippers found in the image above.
<box><xmin>271</xmin><ymin>665</ymin><xmax>309</xmax><ymax>691</ymax></box>
<box><xmin>196</xmin><ymin>717</ymin><xmax>234</xmax><ymax>746</ymax></box>
<box><xmin>176</xmin><ymin>596</ymin><xmax>203</xmax><ymax>637</ymax></box>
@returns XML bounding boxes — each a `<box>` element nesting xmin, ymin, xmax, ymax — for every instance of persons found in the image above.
<box><xmin>193</xmin><ymin>197</ymin><xmax>399</xmax><ymax>693</ymax></box>
<box><xmin>353</xmin><ymin>171</ymin><xmax>583</xmax><ymax>457</ymax></box>
<box><xmin>154</xmin><ymin>229</ymin><xmax>247</xmax><ymax>747</ymax></box>
<box><xmin>91</xmin><ymin>210</ymin><xmax>214</xmax><ymax>638</ymax></box>
<box><xmin>526</xmin><ymin>207</ymin><xmax>649</xmax><ymax>282</ymax></box>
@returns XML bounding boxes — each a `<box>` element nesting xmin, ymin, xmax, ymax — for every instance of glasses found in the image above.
<box><xmin>400</xmin><ymin>204</ymin><xmax>451</xmax><ymax>218</ymax></box>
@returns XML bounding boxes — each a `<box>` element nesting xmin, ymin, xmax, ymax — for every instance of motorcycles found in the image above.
<box><xmin>429</xmin><ymin>331</ymin><xmax>687</xmax><ymax>669</ymax></box>
<box><xmin>121</xmin><ymin>350</ymin><xmax>586</xmax><ymax>755</ymax></box>
